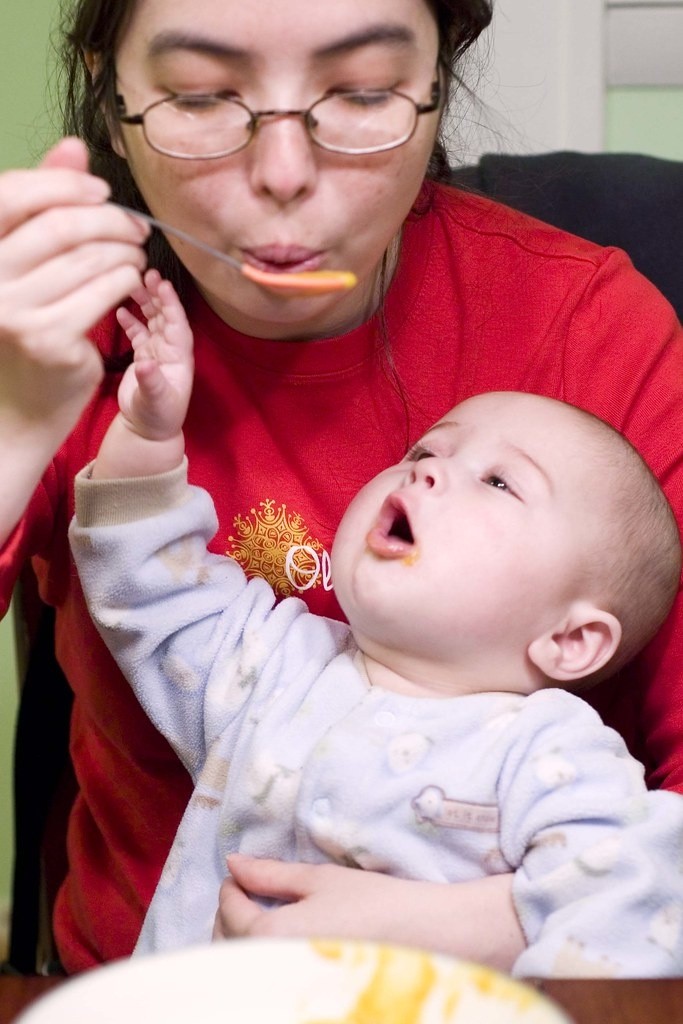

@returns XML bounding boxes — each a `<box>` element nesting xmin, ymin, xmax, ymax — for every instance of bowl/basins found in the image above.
<box><xmin>14</xmin><ymin>940</ymin><xmax>571</xmax><ymax>1024</ymax></box>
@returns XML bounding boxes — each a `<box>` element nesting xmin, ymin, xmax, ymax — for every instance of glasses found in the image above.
<box><xmin>106</xmin><ymin>46</ymin><xmax>440</xmax><ymax>160</ymax></box>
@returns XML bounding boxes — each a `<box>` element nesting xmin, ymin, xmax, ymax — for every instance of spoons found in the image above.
<box><xmin>109</xmin><ymin>202</ymin><xmax>357</xmax><ymax>293</ymax></box>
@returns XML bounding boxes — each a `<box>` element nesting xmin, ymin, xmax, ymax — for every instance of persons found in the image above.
<box><xmin>0</xmin><ymin>2</ymin><xmax>680</xmax><ymax>980</ymax></box>
<box><xmin>68</xmin><ymin>268</ymin><xmax>682</xmax><ymax>979</ymax></box>
